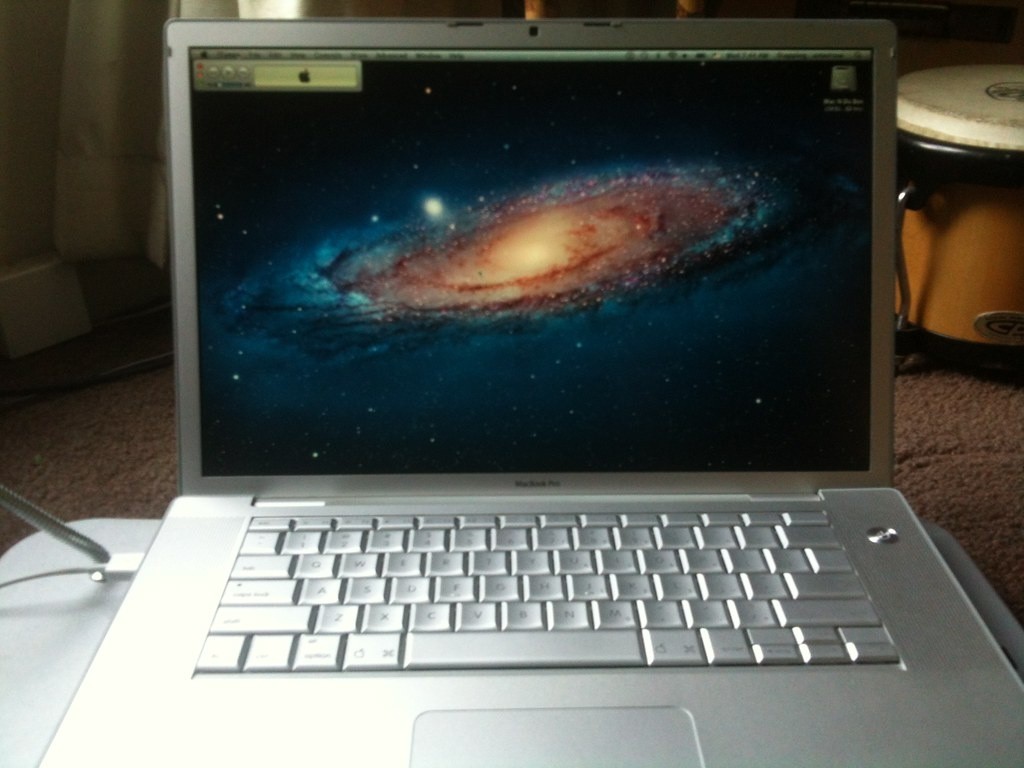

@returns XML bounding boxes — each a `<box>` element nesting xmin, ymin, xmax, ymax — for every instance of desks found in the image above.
<box><xmin>0</xmin><ymin>516</ymin><xmax>1024</xmax><ymax>768</ymax></box>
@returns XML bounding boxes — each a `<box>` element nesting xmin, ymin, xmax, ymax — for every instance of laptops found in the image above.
<box><xmin>35</xmin><ymin>17</ymin><xmax>1024</xmax><ymax>768</ymax></box>
